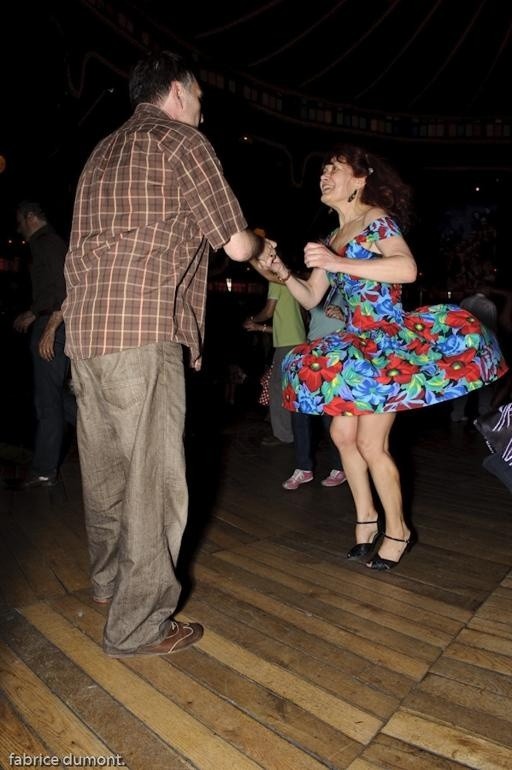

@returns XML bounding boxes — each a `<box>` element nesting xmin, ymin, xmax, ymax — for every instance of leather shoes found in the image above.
<box><xmin>346</xmin><ymin>513</ymin><xmax>386</xmax><ymax>560</ymax></box>
<box><xmin>366</xmin><ymin>527</ymin><xmax>417</xmax><ymax>569</ymax></box>
<box><xmin>104</xmin><ymin>617</ymin><xmax>202</xmax><ymax>655</ymax></box>
<box><xmin>12</xmin><ymin>468</ymin><xmax>57</xmax><ymax>490</ymax></box>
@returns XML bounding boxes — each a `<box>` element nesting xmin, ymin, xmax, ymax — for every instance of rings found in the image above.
<box><xmin>270</xmin><ymin>255</ymin><xmax>273</xmax><ymax>257</ymax></box>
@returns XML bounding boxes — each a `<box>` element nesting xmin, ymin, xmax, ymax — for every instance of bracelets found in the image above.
<box><xmin>263</xmin><ymin>325</ymin><xmax>266</xmax><ymax>332</ymax></box>
<box><xmin>250</xmin><ymin>316</ymin><xmax>254</xmax><ymax>320</ymax></box>
<box><xmin>282</xmin><ymin>272</ymin><xmax>290</xmax><ymax>282</ymax></box>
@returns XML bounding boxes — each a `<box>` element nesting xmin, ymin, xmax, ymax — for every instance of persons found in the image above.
<box><xmin>267</xmin><ymin>144</ymin><xmax>508</xmax><ymax>570</ymax></box>
<box><xmin>282</xmin><ymin>286</ymin><xmax>347</xmax><ymax>489</ymax></box>
<box><xmin>59</xmin><ymin>50</ymin><xmax>277</xmax><ymax>655</ymax></box>
<box><xmin>450</xmin><ymin>280</ymin><xmax>497</xmax><ymax>422</ymax></box>
<box><xmin>243</xmin><ymin>281</ymin><xmax>306</xmax><ymax>447</ymax></box>
<box><xmin>14</xmin><ymin>199</ymin><xmax>68</xmax><ymax>488</ymax></box>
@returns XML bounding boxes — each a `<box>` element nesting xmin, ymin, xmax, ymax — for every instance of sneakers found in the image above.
<box><xmin>321</xmin><ymin>470</ymin><xmax>346</xmax><ymax>487</ymax></box>
<box><xmin>282</xmin><ymin>469</ymin><xmax>314</xmax><ymax>489</ymax></box>
